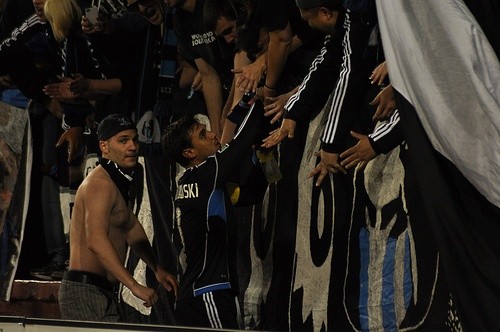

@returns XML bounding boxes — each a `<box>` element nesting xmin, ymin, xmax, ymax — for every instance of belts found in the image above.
<box><xmin>63</xmin><ymin>270</ymin><xmax>118</xmax><ymax>291</ymax></box>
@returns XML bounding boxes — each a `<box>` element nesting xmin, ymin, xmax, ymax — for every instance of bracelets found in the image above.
<box><xmin>265</xmin><ymin>84</ymin><xmax>274</xmax><ymax>90</ymax></box>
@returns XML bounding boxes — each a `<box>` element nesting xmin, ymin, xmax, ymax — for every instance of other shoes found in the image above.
<box><xmin>31</xmin><ymin>270</ymin><xmax>54</xmax><ymax>280</ymax></box>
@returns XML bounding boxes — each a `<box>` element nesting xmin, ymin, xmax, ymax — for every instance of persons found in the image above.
<box><xmin>57</xmin><ymin>113</ymin><xmax>180</xmax><ymax>327</ymax></box>
<box><xmin>0</xmin><ymin>0</ymin><xmax>425</xmax><ymax>281</ymax></box>
<box><xmin>159</xmin><ymin>98</ymin><xmax>269</xmax><ymax>329</ymax></box>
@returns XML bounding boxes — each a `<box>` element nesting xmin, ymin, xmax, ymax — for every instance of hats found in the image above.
<box><xmin>98</xmin><ymin>114</ymin><xmax>136</xmax><ymax>140</ymax></box>
